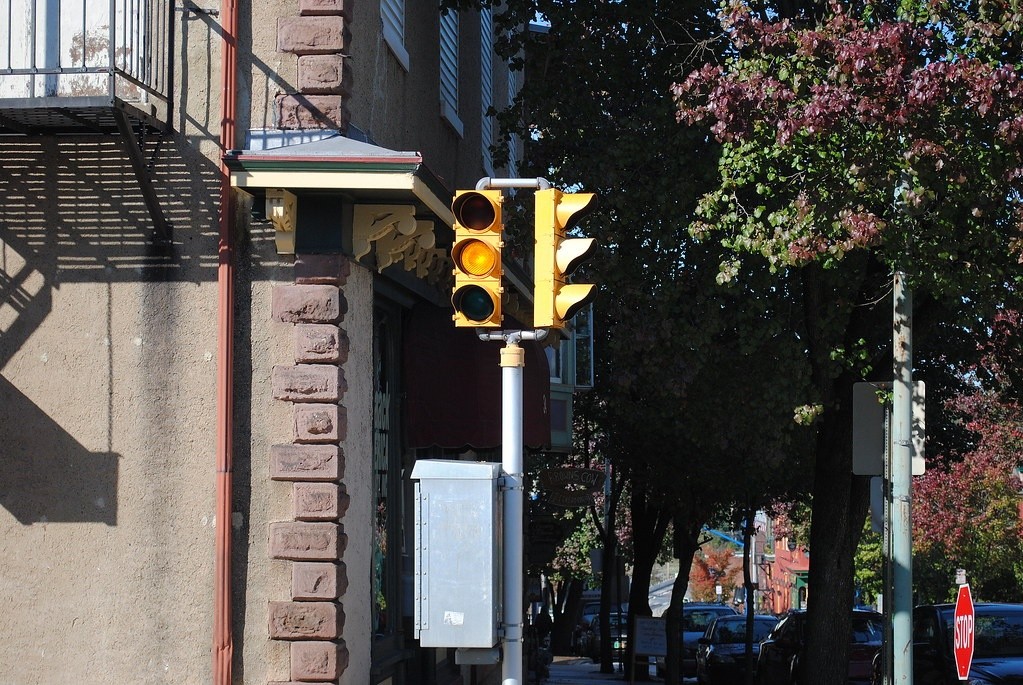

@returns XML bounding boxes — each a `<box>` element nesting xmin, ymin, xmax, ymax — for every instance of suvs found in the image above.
<box><xmin>758</xmin><ymin>606</ymin><xmax>891</xmax><ymax>685</ymax></box>
<box><xmin>656</xmin><ymin>601</ymin><xmax>738</xmax><ymax>680</ymax></box>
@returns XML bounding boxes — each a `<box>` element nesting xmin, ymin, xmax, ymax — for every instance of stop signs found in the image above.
<box><xmin>954</xmin><ymin>583</ymin><xmax>975</xmax><ymax>681</ymax></box>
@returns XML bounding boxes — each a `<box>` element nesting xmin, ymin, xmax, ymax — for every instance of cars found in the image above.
<box><xmin>587</xmin><ymin>611</ymin><xmax>628</xmax><ymax>665</ymax></box>
<box><xmin>574</xmin><ymin>597</ymin><xmax>623</xmax><ymax>652</ymax></box>
<box><xmin>868</xmin><ymin>603</ymin><xmax>1021</xmax><ymax>685</ymax></box>
<box><xmin>696</xmin><ymin>615</ymin><xmax>779</xmax><ymax>684</ymax></box>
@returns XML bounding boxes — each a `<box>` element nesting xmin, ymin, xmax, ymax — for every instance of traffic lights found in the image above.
<box><xmin>528</xmin><ymin>187</ymin><xmax>599</xmax><ymax>329</ymax></box>
<box><xmin>450</xmin><ymin>188</ymin><xmax>504</xmax><ymax>328</ymax></box>
